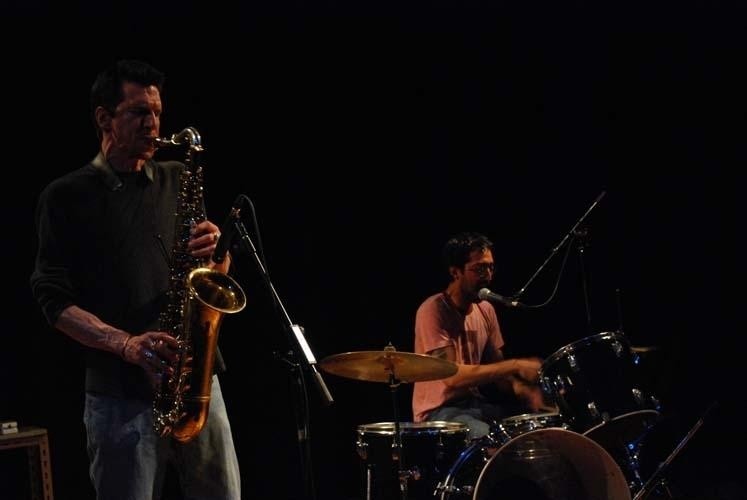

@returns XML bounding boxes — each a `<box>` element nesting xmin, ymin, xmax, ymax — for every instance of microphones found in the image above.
<box><xmin>478</xmin><ymin>288</ymin><xmax>520</xmax><ymax>308</ymax></box>
<box><xmin>212</xmin><ymin>194</ymin><xmax>247</xmax><ymax>265</ymax></box>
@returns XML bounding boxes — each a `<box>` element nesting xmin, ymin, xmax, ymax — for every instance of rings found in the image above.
<box><xmin>144</xmin><ymin>351</ymin><xmax>154</xmax><ymax>361</ymax></box>
<box><xmin>149</xmin><ymin>340</ymin><xmax>161</xmax><ymax>352</ymax></box>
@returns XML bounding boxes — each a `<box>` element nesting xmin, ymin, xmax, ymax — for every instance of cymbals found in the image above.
<box><xmin>318</xmin><ymin>350</ymin><xmax>457</xmax><ymax>382</ymax></box>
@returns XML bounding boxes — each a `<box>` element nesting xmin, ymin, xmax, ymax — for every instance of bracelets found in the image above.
<box><xmin>121</xmin><ymin>335</ymin><xmax>135</xmax><ymax>361</ymax></box>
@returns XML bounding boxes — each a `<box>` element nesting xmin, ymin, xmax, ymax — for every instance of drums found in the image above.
<box><xmin>434</xmin><ymin>424</ymin><xmax>633</xmax><ymax>499</ymax></box>
<box><xmin>538</xmin><ymin>331</ymin><xmax>660</xmax><ymax>446</ymax></box>
<box><xmin>356</xmin><ymin>421</ymin><xmax>472</xmax><ymax>472</ymax></box>
<box><xmin>499</xmin><ymin>412</ymin><xmax>571</xmax><ymax>431</ymax></box>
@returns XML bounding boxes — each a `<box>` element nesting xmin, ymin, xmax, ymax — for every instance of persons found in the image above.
<box><xmin>412</xmin><ymin>230</ymin><xmax>560</xmax><ymax>460</ymax></box>
<box><xmin>28</xmin><ymin>53</ymin><xmax>245</xmax><ymax>500</ymax></box>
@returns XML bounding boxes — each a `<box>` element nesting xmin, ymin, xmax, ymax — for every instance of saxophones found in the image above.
<box><xmin>149</xmin><ymin>127</ymin><xmax>246</xmax><ymax>446</ymax></box>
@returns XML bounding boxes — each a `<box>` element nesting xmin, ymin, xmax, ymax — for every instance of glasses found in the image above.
<box><xmin>465</xmin><ymin>261</ymin><xmax>494</xmax><ymax>275</ymax></box>
